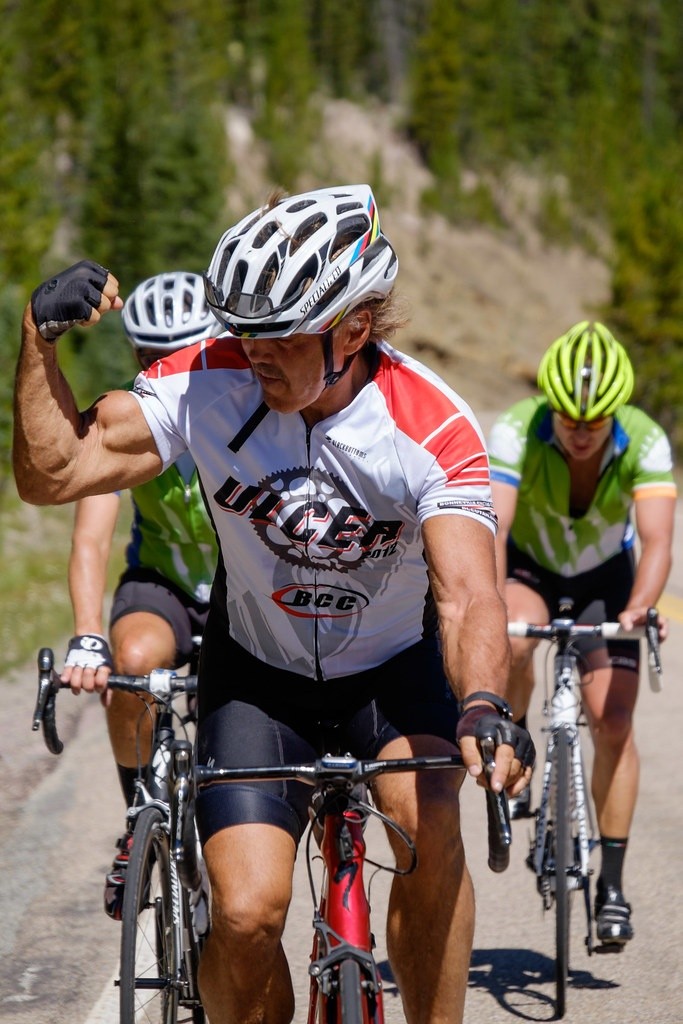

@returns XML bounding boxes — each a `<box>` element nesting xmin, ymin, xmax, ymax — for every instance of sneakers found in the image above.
<box><xmin>104</xmin><ymin>833</ymin><xmax>155</xmax><ymax>920</ymax></box>
<box><xmin>508</xmin><ymin>787</ymin><xmax>530</xmax><ymax>818</ymax></box>
<box><xmin>596</xmin><ymin>889</ymin><xmax>633</xmax><ymax>943</ymax></box>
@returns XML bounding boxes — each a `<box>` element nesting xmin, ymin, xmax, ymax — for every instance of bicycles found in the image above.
<box><xmin>32</xmin><ymin>646</ymin><xmax>205</xmax><ymax>1024</ymax></box>
<box><xmin>508</xmin><ymin>607</ymin><xmax>663</xmax><ymax>1019</ymax></box>
<box><xmin>171</xmin><ymin>722</ymin><xmax>513</xmax><ymax>1024</ymax></box>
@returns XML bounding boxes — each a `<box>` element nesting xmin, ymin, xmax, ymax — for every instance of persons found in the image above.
<box><xmin>63</xmin><ymin>271</ymin><xmax>223</xmax><ymax>921</ymax></box>
<box><xmin>12</xmin><ymin>183</ymin><xmax>537</xmax><ymax>1024</ymax></box>
<box><xmin>487</xmin><ymin>320</ymin><xmax>678</xmax><ymax>939</ymax></box>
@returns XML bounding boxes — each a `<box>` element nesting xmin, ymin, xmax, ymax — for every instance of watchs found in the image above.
<box><xmin>460</xmin><ymin>691</ymin><xmax>513</xmax><ymax>720</ymax></box>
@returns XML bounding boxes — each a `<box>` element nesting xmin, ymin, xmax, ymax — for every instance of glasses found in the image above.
<box><xmin>201</xmin><ymin>270</ymin><xmax>308</xmax><ymax>319</ymax></box>
<box><xmin>555</xmin><ymin>414</ymin><xmax>614</xmax><ymax>431</ymax></box>
<box><xmin>137</xmin><ymin>351</ymin><xmax>164</xmax><ymax>371</ymax></box>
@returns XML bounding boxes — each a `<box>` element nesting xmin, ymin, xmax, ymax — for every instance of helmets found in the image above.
<box><xmin>207</xmin><ymin>184</ymin><xmax>399</xmax><ymax>340</ymax></box>
<box><xmin>537</xmin><ymin>321</ymin><xmax>634</xmax><ymax>421</ymax></box>
<box><xmin>120</xmin><ymin>271</ymin><xmax>222</xmax><ymax>351</ymax></box>
